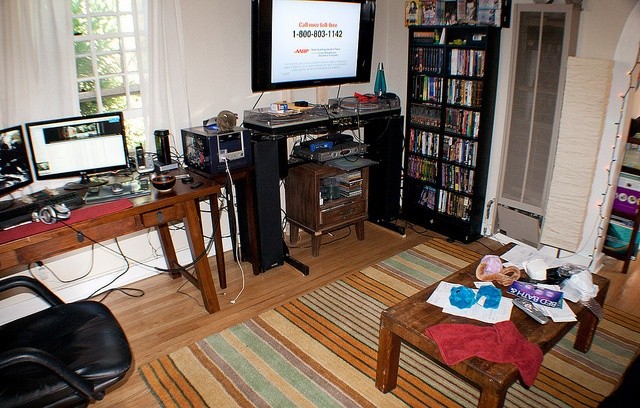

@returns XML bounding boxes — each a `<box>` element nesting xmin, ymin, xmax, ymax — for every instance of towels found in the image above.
<box><xmin>423</xmin><ymin>320</ymin><xmax>544</xmax><ymax>389</ymax></box>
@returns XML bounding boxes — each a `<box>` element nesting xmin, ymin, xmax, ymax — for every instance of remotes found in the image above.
<box><xmin>512</xmin><ymin>297</ymin><xmax>549</xmax><ymax>326</ymax></box>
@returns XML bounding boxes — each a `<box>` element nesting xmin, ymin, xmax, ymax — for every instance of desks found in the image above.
<box><xmin>286</xmin><ymin>158</ymin><xmax>377</xmax><ymax>254</ymax></box>
<box><xmin>0</xmin><ymin>165</ymin><xmax>221</xmax><ymax>315</ymax></box>
<box><xmin>375</xmin><ymin>241</ymin><xmax>611</xmax><ymax>407</ymax></box>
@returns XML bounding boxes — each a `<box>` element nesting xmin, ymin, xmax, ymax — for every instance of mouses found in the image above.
<box><xmin>111</xmin><ymin>183</ymin><xmax>123</xmax><ymax>195</ymax></box>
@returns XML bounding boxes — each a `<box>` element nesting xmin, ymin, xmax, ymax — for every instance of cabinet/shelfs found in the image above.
<box><xmin>401</xmin><ymin>24</ymin><xmax>501</xmax><ymax>244</ymax></box>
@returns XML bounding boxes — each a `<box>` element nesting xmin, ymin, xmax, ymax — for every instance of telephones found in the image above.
<box><xmin>136</xmin><ymin>143</ymin><xmax>154</xmax><ymax>173</ymax></box>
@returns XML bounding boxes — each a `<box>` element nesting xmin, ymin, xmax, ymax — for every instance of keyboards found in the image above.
<box><xmin>0</xmin><ymin>192</ymin><xmax>85</xmax><ymax>231</ymax></box>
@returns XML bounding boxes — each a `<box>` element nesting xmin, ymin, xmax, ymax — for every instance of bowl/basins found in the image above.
<box><xmin>152</xmin><ymin>175</ymin><xmax>177</xmax><ymax>192</ymax></box>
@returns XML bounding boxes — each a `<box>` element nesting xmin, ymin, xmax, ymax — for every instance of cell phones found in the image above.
<box><xmin>86</xmin><ymin>186</ymin><xmax>100</xmax><ymax>197</ymax></box>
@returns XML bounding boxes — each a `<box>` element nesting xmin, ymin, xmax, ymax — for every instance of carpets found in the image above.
<box><xmin>138</xmin><ymin>237</ymin><xmax>640</xmax><ymax>407</ymax></box>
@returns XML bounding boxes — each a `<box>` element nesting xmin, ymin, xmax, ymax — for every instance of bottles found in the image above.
<box><xmin>153</xmin><ymin>129</ymin><xmax>173</xmax><ymax>165</ymax></box>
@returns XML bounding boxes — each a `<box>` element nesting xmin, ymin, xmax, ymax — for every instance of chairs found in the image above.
<box><xmin>0</xmin><ymin>274</ymin><xmax>132</xmax><ymax>407</ymax></box>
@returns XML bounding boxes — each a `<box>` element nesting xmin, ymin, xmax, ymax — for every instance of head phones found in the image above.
<box><xmin>32</xmin><ymin>203</ymin><xmax>71</xmax><ymax>226</ymax></box>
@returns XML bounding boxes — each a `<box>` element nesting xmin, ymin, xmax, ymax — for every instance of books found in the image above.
<box><xmin>442</xmin><ymin>163</ymin><xmax>475</xmax><ymax>195</ymax></box>
<box><xmin>417</xmin><ymin>186</ymin><xmax>436</xmax><ymax>211</ymax></box>
<box><xmin>410</xmin><ymin>128</ymin><xmax>440</xmax><ymax>158</ymax></box>
<box><xmin>442</xmin><ymin>135</ymin><xmax>479</xmax><ymax>167</ymax></box>
<box><xmin>444</xmin><ymin>109</ymin><xmax>481</xmax><ymax>140</ymax></box>
<box><xmin>408</xmin><ymin>155</ymin><xmax>438</xmax><ymax>184</ymax></box>
<box><xmin>404</xmin><ymin>0</ymin><xmax>512</xmax><ymax>29</ymax></box>
<box><xmin>410</xmin><ymin>102</ymin><xmax>442</xmax><ymax>128</ymax></box>
<box><xmin>438</xmin><ymin>189</ymin><xmax>472</xmax><ymax>222</ymax></box>
<box><xmin>448</xmin><ymin>48</ymin><xmax>486</xmax><ymax>77</ymax></box>
<box><xmin>411</xmin><ymin>76</ymin><xmax>444</xmax><ymax>102</ymax></box>
<box><xmin>447</xmin><ymin>79</ymin><xmax>483</xmax><ymax>108</ymax></box>
<box><xmin>410</xmin><ymin>45</ymin><xmax>444</xmax><ymax>74</ymax></box>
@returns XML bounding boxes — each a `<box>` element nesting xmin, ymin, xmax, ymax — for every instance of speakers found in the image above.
<box><xmin>365</xmin><ymin>116</ymin><xmax>404</xmax><ymax>222</ymax></box>
<box><xmin>234</xmin><ymin>137</ymin><xmax>284</xmax><ymax>273</ymax></box>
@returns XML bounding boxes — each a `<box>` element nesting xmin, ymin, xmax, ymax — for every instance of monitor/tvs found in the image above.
<box><xmin>251</xmin><ymin>0</ymin><xmax>376</xmax><ymax>94</ymax></box>
<box><xmin>24</xmin><ymin>111</ymin><xmax>130</xmax><ymax>189</ymax></box>
<box><xmin>0</xmin><ymin>125</ymin><xmax>34</xmax><ymax>211</ymax></box>
